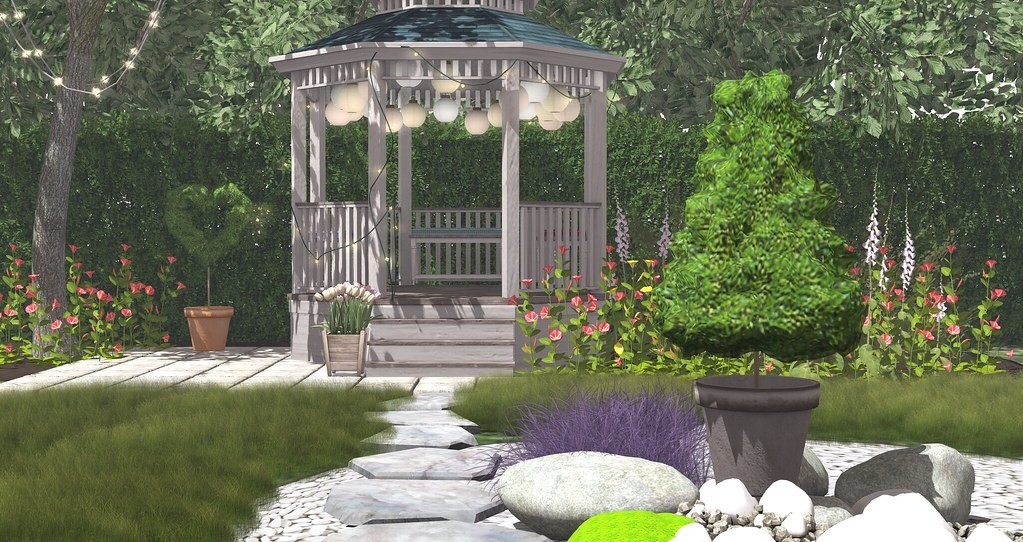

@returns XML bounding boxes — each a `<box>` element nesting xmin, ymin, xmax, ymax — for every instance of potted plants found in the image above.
<box><xmin>164</xmin><ymin>182</ymin><xmax>253</xmax><ymax>351</ymax></box>
<box><xmin>321</xmin><ymin>328</ymin><xmax>367</xmax><ymax>376</ymax></box>
<box><xmin>650</xmin><ymin>69</ymin><xmax>869</xmax><ymax>496</ymax></box>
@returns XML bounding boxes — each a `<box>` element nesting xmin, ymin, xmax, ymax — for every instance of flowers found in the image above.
<box><xmin>0</xmin><ymin>242</ymin><xmax>51</xmax><ymax>359</ymax></box>
<box><xmin>309</xmin><ymin>282</ymin><xmax>384</xmax><ymax>334</ymax></box>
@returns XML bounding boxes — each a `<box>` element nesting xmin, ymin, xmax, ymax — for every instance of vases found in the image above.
<box><xmin>0</xmin><ymin>359</ymin><xmax>39</xmax><ymax>381</ymax></box>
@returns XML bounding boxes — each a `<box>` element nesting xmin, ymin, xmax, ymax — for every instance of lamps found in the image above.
<box><xmin>431</xmin><ymin>61</ymin><xmax>462</xmax><ymax>94</ymax></box>
<box><xmin>519</xmin><ymin>70</ymin><xmax>580</xmax><ymax>130</ymax></box>
<box><xmin>433</xmin><ymin>95</ymin><xmax>459</xmax><ymax>123</ymax></box>
<box><xmin>325</xmin><ymin>80</ymin><xmax>368</xmax><ymax>126</ymax></box>
<box><xmin>385</xmin><ymin>105</ymin><xmax>402</xmax><ymax>133</ymax></box>
<box><xmin>464</xmin><ymin>107</ymin><xmax>489</xmax><ymax>135</ymax></box>
<box><xmin>401</xmin><ymin>100</ymin><xmax>426</xmax><ymax>128</ymax></box>
<box><xmin>394</xmin><ymin>61</ymin><xmax>424</xmax><ymax>88</ymax></box>
<box><xmin>487</xmin><ymin>100</ymin><xmax>502</xmax><ymax>127</ymax></box>
